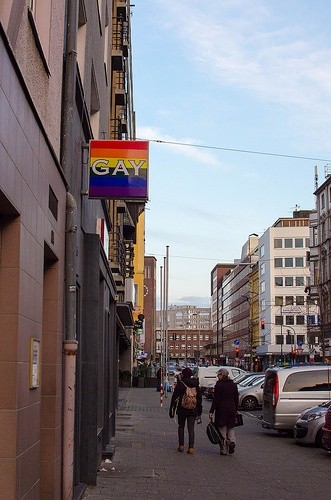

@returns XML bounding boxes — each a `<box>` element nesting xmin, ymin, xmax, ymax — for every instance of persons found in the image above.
<box><xmin>157</xmin><ymin>368</ymin><xmax>161</xmax><ymax>392</ymax></box>
<box><xmin>177</xmin><ymin>373</ymin><xmax>182</xmax><ymax>383</ymax></box>
<box><xmin>254</xmin><ymin>364</ymin><xmax>258</xmax><ymax>372</ymax></box>
<box><xmin>209</xmin><ymin>368</ymin><xmax>239</xmax><ymax>456</ymax></box>
<box><xmin>169</xmin><ymin>368</ymin><xmax>202</xmax><ymax>454</ymax></box>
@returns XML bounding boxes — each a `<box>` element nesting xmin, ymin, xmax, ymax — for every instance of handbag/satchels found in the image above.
<box><xmin>234</xmin><ymin>412</ymin><xmax>243</xmax><ymax>426</ymax></box>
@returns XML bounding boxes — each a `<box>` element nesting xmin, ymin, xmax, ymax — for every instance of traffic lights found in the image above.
<box><xmin>236</xmin><ymin>348</ymin><xmax>238</xmax><ymax>357</ymax></box>
<box><xmin>261</xmin><ymin>321</ymin><xmax>264</xmax><ymax>329</ymax></box>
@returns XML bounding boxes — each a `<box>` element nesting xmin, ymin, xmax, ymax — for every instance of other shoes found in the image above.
<box><xmin>220</xmin><ymin>451</ymin><xmax>227</xmax><ymax>454</ymax></box>
<box><xmin>178</xmin><ymin>446</ymin><xmax>184</xmax><ymax>451</ymax></box>
<box><xmin>188</xmin><ymin>447</ymin><xmax>194</xmax><ymax>454</ymax></box>
<box><xmin>229</xmin><ymin>443</ymin><xmax>235</xmax><ymax>454</ymax></box>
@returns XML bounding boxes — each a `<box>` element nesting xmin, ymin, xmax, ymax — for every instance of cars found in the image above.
<box><xmin>168</xmin><ymin>365</ymin><xmax>175</xmax><ymax>375</ymax></box>
<box><xmin>293</xmin><ymin>400</ymin><xmax>331</xmax><ymax>452</ymax></box>
<box><xmin>232</xmin><ymin>372</ymin><xmax>266</xmax><ymax>412</ymax></box>
<box><xmin>204</xmin><ymin>383</ymin><xmax>216</xmax><ymax>398</ymax></box>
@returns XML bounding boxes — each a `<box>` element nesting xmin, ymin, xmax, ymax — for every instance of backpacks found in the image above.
<box><xmin>207</xmin><ymin>417</ymin><xmax>225</xmax><ymax>444</ymax></box>
<box><xmin>178</xmin><ymin>381</ymin><xmax>197</xmax><ymax>410</ymax></box>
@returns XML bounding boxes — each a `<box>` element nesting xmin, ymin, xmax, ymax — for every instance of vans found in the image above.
<box><xmin>258</xmin><ymin>365</ymin><xmax>331</xmax><ymax>431</ymax></box>
<box><xmin>196</xmin><ymin>366</ymin><xmax>247</xmax><ymax>395</ymax></box>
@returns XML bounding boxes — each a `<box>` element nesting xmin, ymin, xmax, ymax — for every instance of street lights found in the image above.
<box><xmin>180</xmin><ymin>322</ymin><xmax>190</xmax><ymax>368</ymax></box>
